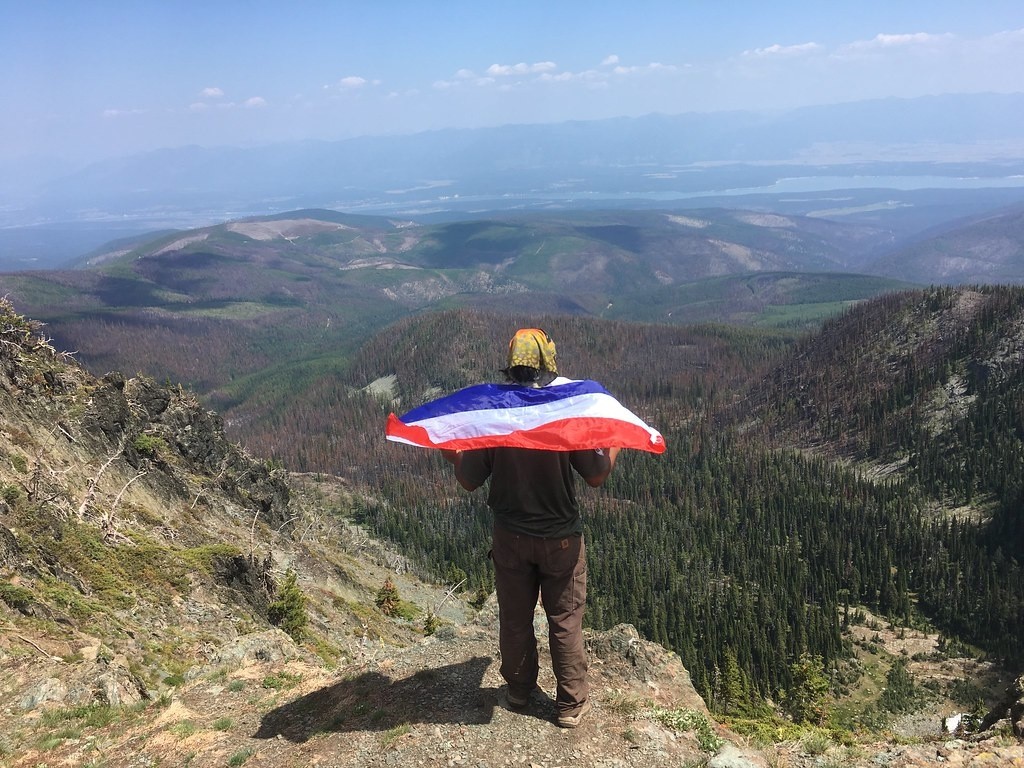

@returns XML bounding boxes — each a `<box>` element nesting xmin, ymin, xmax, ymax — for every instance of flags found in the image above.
<box><xmin>387</xmin><ymin>376</ymin><xmax>668</xmax><ymax>453</ymax></box>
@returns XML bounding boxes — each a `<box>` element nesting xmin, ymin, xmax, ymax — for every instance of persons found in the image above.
<box><xmin>440</xmin><ymin>328</ymin><xmax>620</xmax><ymax>728</ymax></box>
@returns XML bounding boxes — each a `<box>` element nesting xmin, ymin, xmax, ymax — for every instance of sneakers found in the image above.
<box><xmin>506</xmin><ymin>682</ymin><xmax>531</xmax><ymax>709</ymax></box>
<box><xmin>557</xmin><ymin>697</ymin><xmax>592</xmax><ymax>729</ymax></box>
